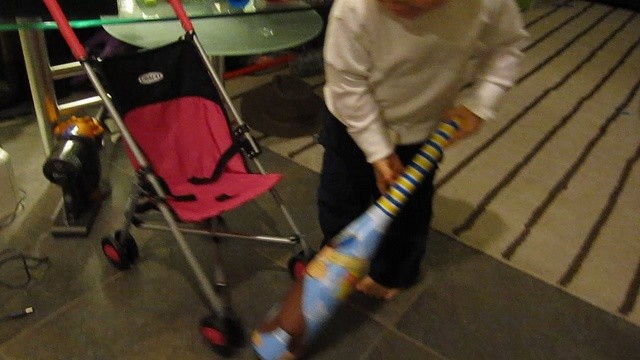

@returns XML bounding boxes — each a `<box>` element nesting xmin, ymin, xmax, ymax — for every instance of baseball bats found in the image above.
<box><xmin>250</xmin><ymin>116</ymin><xmax>463</xmax><ymax>359</ymax></box>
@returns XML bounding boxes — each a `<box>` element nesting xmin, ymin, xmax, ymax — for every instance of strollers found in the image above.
<box><xmin>37</xmin><ymin>0</ymin><xmax>319</xmax><ymax>356</ymax></box>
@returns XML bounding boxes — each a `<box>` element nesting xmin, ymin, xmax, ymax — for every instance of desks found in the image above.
<box><xmin>99</xmin><ymin>0</ymin><xmax>324</xmax><ymax>130</ymax></box>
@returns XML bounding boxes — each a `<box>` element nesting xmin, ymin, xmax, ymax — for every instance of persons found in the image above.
<box><xmin>315</xmin><ymin>2</ymin><xmax>527</xmax><ymax>309</ymax></box>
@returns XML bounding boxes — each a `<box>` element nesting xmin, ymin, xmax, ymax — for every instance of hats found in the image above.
<box><xmin>240</xmin><ymin>74</ymin><xmax>325</xmax><ymax>138</ymax></box>
